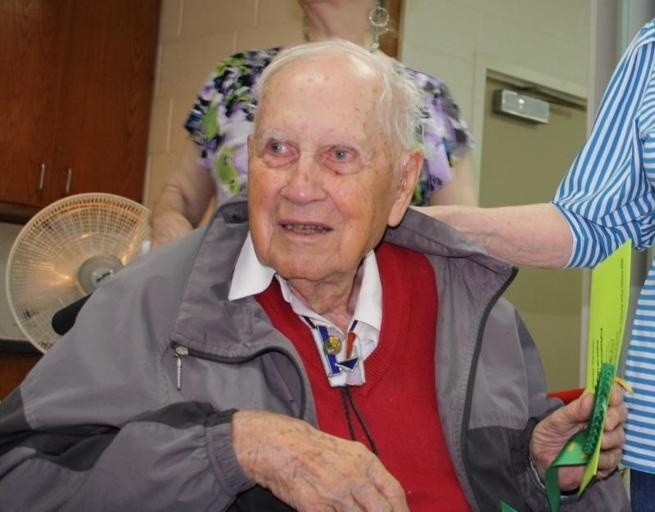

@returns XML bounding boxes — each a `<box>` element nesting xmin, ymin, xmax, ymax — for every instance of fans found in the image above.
<box><xmin>4</xmin><ymin>192</ymin><xmax>153</xmax><ymax>357</ymax></box>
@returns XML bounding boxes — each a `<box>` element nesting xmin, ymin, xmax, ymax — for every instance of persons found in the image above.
<box><xmin>414</xmin><ymin>20</ymin><xmax>655</xmax><ymax>512</ymax></box>
<box><xmin>148</xmin><ymin>0</ymin><xmax>479</xmax><ymax>247</ymax></box>
<box><xmin>0</xmin><ymin>36</ymin><xmax>629</xmax><ymax>512</ymax></box>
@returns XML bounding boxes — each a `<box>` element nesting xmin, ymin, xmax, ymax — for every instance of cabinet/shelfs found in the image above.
<box><xmin>1</xmin><ymin>0</ymin><xmax>161</xmax><ymax>208</ymax></box>
